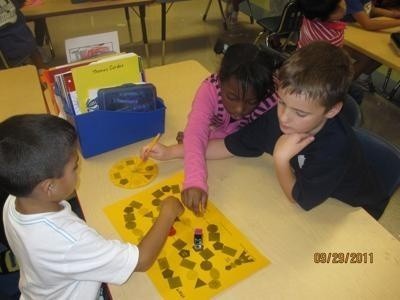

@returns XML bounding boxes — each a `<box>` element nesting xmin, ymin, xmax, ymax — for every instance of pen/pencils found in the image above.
<box><xmin>133</xmin><ymin>131</ymin><xmax>162</xmax><ymax>173</ymax></box>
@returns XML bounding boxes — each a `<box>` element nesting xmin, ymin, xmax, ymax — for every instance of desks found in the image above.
<box><xmin>336</xmin><ymin>14</ymin><xmax>400</xmax><ymax>92</ymax></box>
<box><xmin>18</xmin><ymin>0</ymin><xmax>175</xmax><ymax>55</ymax></box>
<box><xmin>42</xmin><ymin>54</ymin><xmax>400</xmax><ymax>300</ymax></box>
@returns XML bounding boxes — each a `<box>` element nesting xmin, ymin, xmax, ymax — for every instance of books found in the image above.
<box><xmin>43</xmin><ymin>28</ymin><xmax>145</xmax><ymax>114</ymax></box>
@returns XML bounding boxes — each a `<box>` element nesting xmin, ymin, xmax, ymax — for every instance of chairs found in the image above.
<box><xmin>252</xmin><ymin>1</ymin><xmax>307</xmax><ymax>49</ymax></box>
<box><xmin>338</xmin><ymin>91</ymin><xmax>399</xmax><ymax>224</ymax></box>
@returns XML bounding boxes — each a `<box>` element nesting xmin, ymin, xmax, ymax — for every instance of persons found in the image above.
<box><xmin>295</xmin><ymin>0</ymin><xmax>363</xmax><ymax>106</ymax></box>
<box><xmin>345</xmin><ymin>0</ymin><xmax>400</xmax><ymax>92</ymax></box>
<box><xmin>0</xmin><ymin>0</ymin><xmax>43</xmax><ymax>71</ymax></box>
<box><xmin>180</xmin><ymin>42</ymin><xmax>280</xmax><ymax>217</ymax></box>
<box><xmin>1</xmin><ymin>114</ymin><xmax>185</xmax><ymax>300</ymax></box>
<box><xmin>141</xmin><ymin>40</ymin><xmax>400</xmax><ymax>219</ymax></box>
